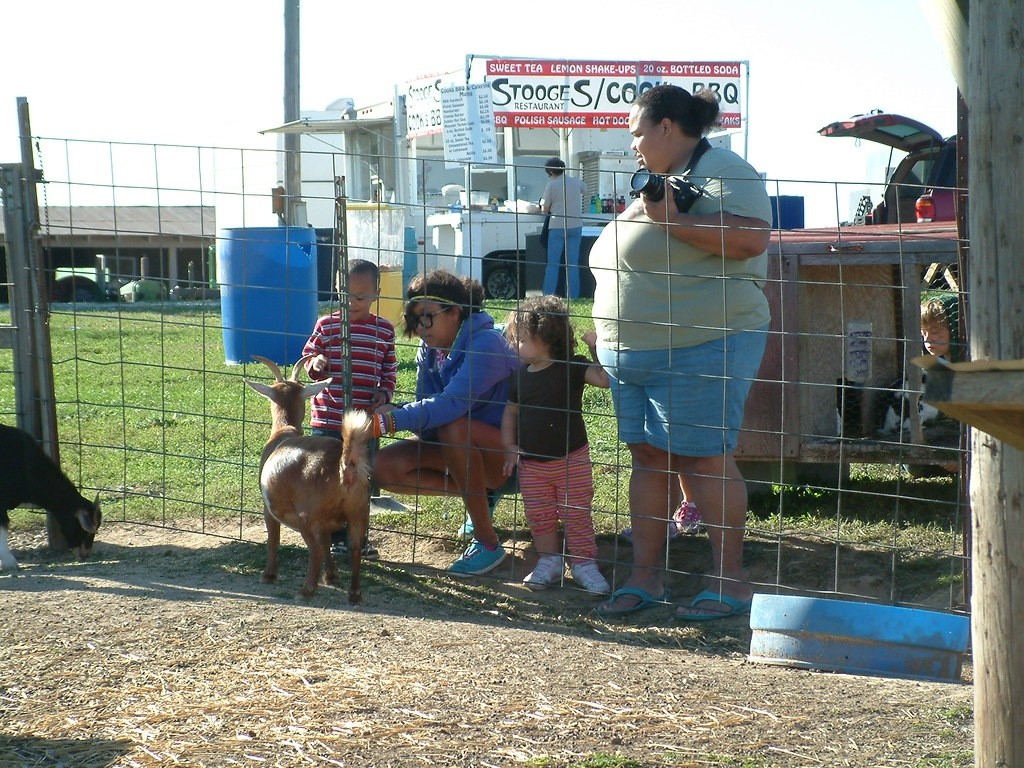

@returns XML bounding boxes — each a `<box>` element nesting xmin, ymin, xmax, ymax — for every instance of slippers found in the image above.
<box><xmin>675</xmin><ymin>590</ymin><xmax>751</xmax><ymax>618</ymax></box>
<box><xmin>598</xmin><ymin>585</ymin><xmax>672</xmax><ymax>615</ymax></box>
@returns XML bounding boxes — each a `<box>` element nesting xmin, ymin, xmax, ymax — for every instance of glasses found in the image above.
<box><xmin>403</xmin><ymin>307</ymin><xmax>451</xmax><ymax>329</ymax></box>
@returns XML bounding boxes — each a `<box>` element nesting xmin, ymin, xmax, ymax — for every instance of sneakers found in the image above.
<box><xmin>571</xmin><ymin>560</ymin><xmax>608</xmax><ymax>593</ymax></box>
<box><xmin>327</xmin><ymin>534</ymin><xmax>379</xmax><ymax>558</ymax></box>
<box><xmin>622</xmin><ymin>520</ymin><xmax>679</xmax><ymax>545</ymax></box>
<box><xmin>447</xmin><ymin>539</ymin><xmax>505</xmax><ymax>578</ymax></box>
<box><xmin>522</xmin><ymin>554</ymin><xmax>567</xmax><ymax>589</ymax></box>
<box><xmin>674</xmin><ymin>499</ymin><xmax>707</xmax><ymax>533</ymax></box>
<box><xmin>455</xmin><ymin>488</ymin><xmax>501</xmax><ymax>537</ymax></box>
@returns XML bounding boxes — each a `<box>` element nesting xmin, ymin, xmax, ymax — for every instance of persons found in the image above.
<box><xmin>588</xmin><ymin>83</ymin><xmax>771</xmax><ymax>621</ymax></box>
<box><xmin>501</xmin><ymin>295</ymin><xmax>612</xmax><ymax>596</ymax></box>
<box><xmin>541</xmin><ymin>158</ymin><xmax>589</xmax><ymax>299</ymax></box>
<box><xmin>369</xmin><ymin>267</ymin><xmax>522</xmax><ymax>578</ymax></box>
<box><xmin>301</xmin><ymin>259</ymin><xmax>397</xmax><ymax>563</ymax></box>
<box><xmin>920</xmin><ymin>294</ymin><xmax>966</xmax><ymax>364</ymax></box>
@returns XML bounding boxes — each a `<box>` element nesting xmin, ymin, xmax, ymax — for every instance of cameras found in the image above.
<box><xmin>631</xmin><ymin>167</ymin><xmax>702</xmax><ymax>213</ymax></box>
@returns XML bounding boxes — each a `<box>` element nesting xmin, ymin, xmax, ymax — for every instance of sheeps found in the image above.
<box><xmin>241</xmin><ymin>353</ymin><xmax>377</xmax><ymax>606</ymax></box>
<box><xmin>0</xmin><ymin>424</ymin><xmax>103</xmax><ymax>578</ymax></box>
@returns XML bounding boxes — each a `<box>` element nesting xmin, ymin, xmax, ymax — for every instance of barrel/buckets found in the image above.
<box><xmin>219</xmin><ymin>225</ymin><xmax>317</xmax><ymax>367</ymax></box>
<box><xmin>369</xmin><ymin>265</ymin><xmax>403</xmax><ymax>333</ymax></box>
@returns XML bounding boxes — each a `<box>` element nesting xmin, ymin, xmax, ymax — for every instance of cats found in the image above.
<box><xmin>837</xmin><ymin>370</ymin><xmax>939</xmax><ymax>444</ymax></box>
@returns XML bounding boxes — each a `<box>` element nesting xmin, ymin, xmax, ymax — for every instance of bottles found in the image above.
<box><xmin>614</xmin><ymin>194</ymin><xmax>620</xmax><ymax>212</ymax></box>
<box><xmin>491</xmin><ymin>196</ymin><xmax>498</xmax><ymax>213</ymax></box>
<box><xmin>608</xmin><ymin>194</ymin><xmax>614</xmax><ymax>213</ymax></box>
<box><xmin>915</xmin><ymin>194</ymin><xmax>935</xmax><ymax>223</ymax></box>
<box><xmin>601</xmin><ymin>193</ymin><xmax>608</xmax><ymax>214</ymax></box>
<box><xmin>596</xmin><ymin>193</ymin><xmax>602</xmax><ymax>214</ymax></box>
<box><xmin>589</xmin><ymin>191</ymin><xmax>596</xmax><ymax>213</ymax></box>
<box><xmin>620</xmin><ymin>195</ymin><xmax>625</xmax><ymax>213</ymax></box>
